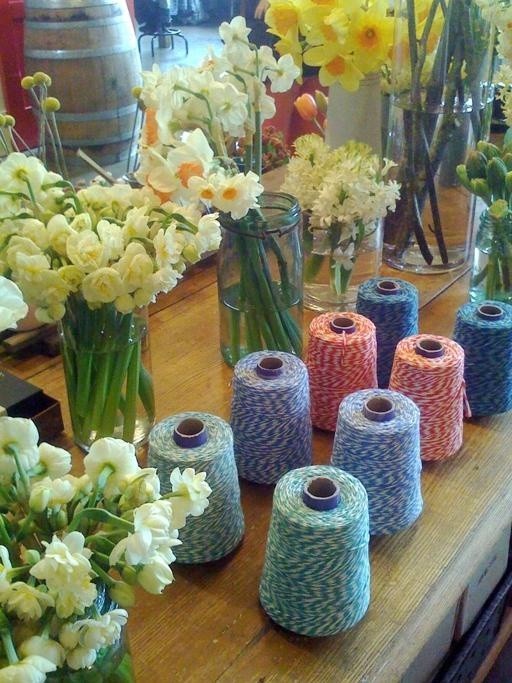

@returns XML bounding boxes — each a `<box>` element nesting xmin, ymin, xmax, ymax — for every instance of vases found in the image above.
<box><xmin>53</xmin><ymin>322</ymin><xmax>157</xmax><ymax>452</ymax></box>
<box><xmin>215</xmin><ymin>192</ymin><xmax>306</xmax><ymax>365</ymax></box>
<box><xmin>299</xmin><ymin>209</ymin><xmax>383</xmax><ymax>314</ymax></box>
<box><xmin>385</xmin><ymin>87</ymin><xmax>494</xmax><ymax>274</ymax></box>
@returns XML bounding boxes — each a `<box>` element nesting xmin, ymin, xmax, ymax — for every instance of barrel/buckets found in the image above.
<box><xmin>22</xmin><ymin>0</ymin><xmax>143</xmax><ymax>174</ymax></box>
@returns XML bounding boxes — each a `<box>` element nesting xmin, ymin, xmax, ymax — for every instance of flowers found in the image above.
<box><xmin>0</xmin><ymin>1</ymin><xmax>512</xmax><ymax>325</ymax></box>
<box><xmin>2</xmin><ymin>414</ymin><xmax>213</xmax><ymax>683</ymax></box>
